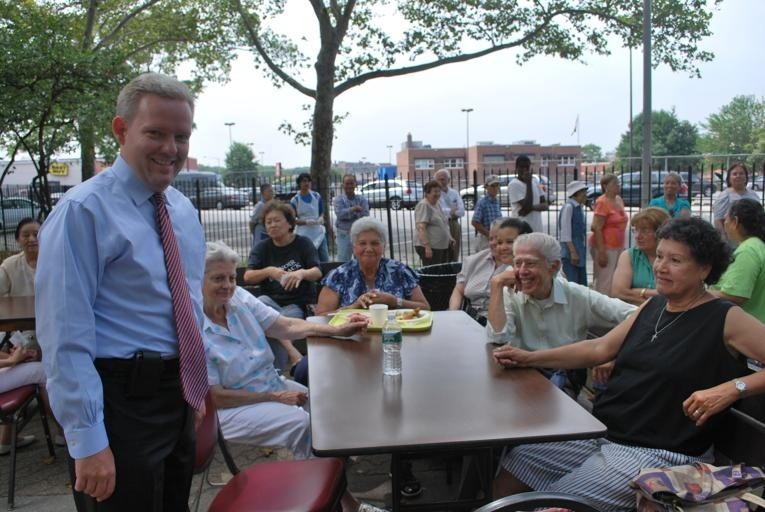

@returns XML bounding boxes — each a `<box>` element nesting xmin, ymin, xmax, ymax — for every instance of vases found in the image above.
<box><xmin>412</xmin><ymin>261</ymin><xmax>463</xmax><ymax>311</ymax></box>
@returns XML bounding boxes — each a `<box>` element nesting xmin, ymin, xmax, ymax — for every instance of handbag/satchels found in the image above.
<box><xmin>634</xmin><ymin>461</ymin><xmax>765</xmax><ymax>512</ymax></box>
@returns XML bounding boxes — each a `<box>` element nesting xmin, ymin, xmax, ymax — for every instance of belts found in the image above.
<box><xmin>466</xmin><ymin>307</ymin><xmax>487</xmax><ymax>327</ymax></box>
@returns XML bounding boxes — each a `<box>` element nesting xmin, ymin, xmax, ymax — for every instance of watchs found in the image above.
<box><xmin>732</xmin><ymin>378</ymin><xmax>746</xmax><ymax>400</ymax></box>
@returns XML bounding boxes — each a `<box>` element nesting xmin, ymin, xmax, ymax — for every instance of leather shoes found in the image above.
<box><xmin>0</xmin><ymin>435</ymin><xmax>34</xmax><ymax>454</ymax></box>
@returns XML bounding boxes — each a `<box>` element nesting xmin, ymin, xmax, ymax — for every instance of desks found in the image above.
<box><xmin>0</xmin><ymin>296</ymin><xmax>36</xmax><ymax>331</ymax></box>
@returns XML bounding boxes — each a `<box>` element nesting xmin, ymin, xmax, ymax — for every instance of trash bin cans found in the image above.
<box><xmin>416</xmin><ymin>262</ymin><xmax>461</xmax><ymax>310</ymax></box>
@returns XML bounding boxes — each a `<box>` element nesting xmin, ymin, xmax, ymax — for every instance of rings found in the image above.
<box><xmin>694</xmin><ymin>408</ymin><xmax>702</xmax><ymax>417</ymax></box>
<box><xmin>497</xmin><ymin>346</ymin><xmax>502</xmax><ymax>352</ymax></box>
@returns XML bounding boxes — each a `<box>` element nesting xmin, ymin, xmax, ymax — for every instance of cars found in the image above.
<box><xmin>677</xmin><ymin>169</ymin><xmax>764</xmax><ymax>198</ymax></box>
<box><xmin>186</xmin><ymin>179</ymin><xmax>299</xmax><ymax>210</ymax></box>
<box><xmin>0</xmin><ymin>195</ymin><xmax>57</xmax><ymax>231</ymax></box>
<box><xmin>49</xmin><ymin>192</ymin><xmax>64</xmax><ymax>205</ymax></box>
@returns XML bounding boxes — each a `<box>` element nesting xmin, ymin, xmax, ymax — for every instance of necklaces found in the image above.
<box><xmin>650</xmin><ymin>291</ymin><xmax>705</xmax><ymax>342</ymax></box>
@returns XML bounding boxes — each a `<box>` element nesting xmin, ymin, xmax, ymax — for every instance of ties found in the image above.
<box><xmin>155</xmin><ymin>192</ymin><xmax>210</xmax><ymax>412</ymax></box>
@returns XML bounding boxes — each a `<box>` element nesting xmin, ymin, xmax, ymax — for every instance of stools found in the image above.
<box><xmin>0</xmin><ymin>382</ymin><xmax>54</xmax><ymax>511</ymax></box>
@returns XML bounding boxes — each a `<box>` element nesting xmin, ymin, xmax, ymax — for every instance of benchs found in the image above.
<box><xmin>558</xmin><ymin>327</ymin><xmax>763</xmax><ymax>468</ymax></box>
<box><xmin>232</xmin><ymin>261</ymin><xmax>348</xmax><ymax>311</ymax></box>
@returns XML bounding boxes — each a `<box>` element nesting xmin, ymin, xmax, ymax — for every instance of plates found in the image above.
<box><xmin>388</xmin><ymin>308</ymin><xmax>429</xmax><ymax>322</ymax></box>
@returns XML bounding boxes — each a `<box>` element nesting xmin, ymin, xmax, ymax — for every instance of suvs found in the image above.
<box><xmin>584</xmin><ymin>170</ymin><xmax>688</xmax><ymax>210</ymax></box>
<box><xmin>332</xmin><ymin>178</ymin><xmax>423</xmax><ymax>211</ymax></box>
<box><xmin>460</xmin><ymin>173</ymin><xmax>555</xmax><ymax>211</ymax></box>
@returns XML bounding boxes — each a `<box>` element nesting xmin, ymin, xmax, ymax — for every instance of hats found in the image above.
<box><xmin>485</xmin><ymin>176</ymin><xmax>500</xmax><ymax>186</ymax></box>
<box><xmin>567</xmin><ymin>182</ymin><xmax>588</xmax><ymax>197</ymax></box>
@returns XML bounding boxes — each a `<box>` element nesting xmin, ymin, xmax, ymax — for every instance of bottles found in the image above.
<box><xmin>380</xmin><ymin>311</ymin><xmax>404</xmax><ymax>374</ymax></box>
<box><xmin>381</xmin><ymin>374</ymin><xmax>404</xmax><ymax>437</ymax></box>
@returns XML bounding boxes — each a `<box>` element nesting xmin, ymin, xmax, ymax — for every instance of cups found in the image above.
<box><xmin>369</xmin><ymin>302</ymin><xmax>388</xmax><ymax>325</ymax></box>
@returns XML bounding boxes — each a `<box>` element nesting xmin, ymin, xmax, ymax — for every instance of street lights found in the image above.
<box><xmin>246</xmin><ymin>143</ymin><xmax>254</xmax><ymax>150</ymax></box>
<box><xmin>223</xmin><ymin>122</ymin><xmax>235</xmax><ymax>170</ymax></box>
<box><xmin>461</xmin><ymin>108</ymin><xmax>474</xmax><ymax>173</ymax></box>
<box><xmin>386</xmin><ymin>145</ymin><xmax>393</xmax><ymax>165</ymax></box>
<box><xmin>257</xmin><ymin>151</ymin><xmax>265</xmax><ymax>167</ymax></box>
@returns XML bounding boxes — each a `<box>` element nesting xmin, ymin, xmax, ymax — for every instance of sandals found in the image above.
<box><xmin>389</xmin><ymin>465</ymin><xmax>422</xmax><ymax>497</ymax></box>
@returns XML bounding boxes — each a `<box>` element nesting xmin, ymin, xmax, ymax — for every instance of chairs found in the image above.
<box><xmin>470</xmin><ymin>485</ymin><xmax>601</xmax><ymax>512</ymax></box>
<box><xmin>180</xmin><ymin>377</ymin><xmax>352</xmax><ymax>512</ymax></box>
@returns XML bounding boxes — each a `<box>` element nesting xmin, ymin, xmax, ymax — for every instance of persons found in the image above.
<box><xmin>242</xmin><ymin>174</ymin><xmax>429</xmax><ymax>323</ymax></box>
<box><xmin>34</xmin><ymin>72</ymin><xmax>206</xmax><ymax>512</ymax></box>
<box><xmin>0</xmin><ymin>217</ymin><xmax>44</xmax><ymax>297</ymax></box>
<box><xmin>0</xmin><ymin>342</ymin><xmax>67</xmax><ymax>455</ymax></box>
<box><xmin>415</xmin><ymin>156</ymin><xmax>764</xmax><ymax>323</ymax></box>
<box><xmin>202</xmin><ymin>239</ymin><xmax>389</xmax><ymax>512</ymax></box>
<box><xmin>485</xmin><ymin>231</ymin><xmax>639</xmax><ymax>385</ymax></box>
<box><xmin>492</xmin><ymin>214</ymin><xmax>764</xmax><ymax>512</ymax></box>
